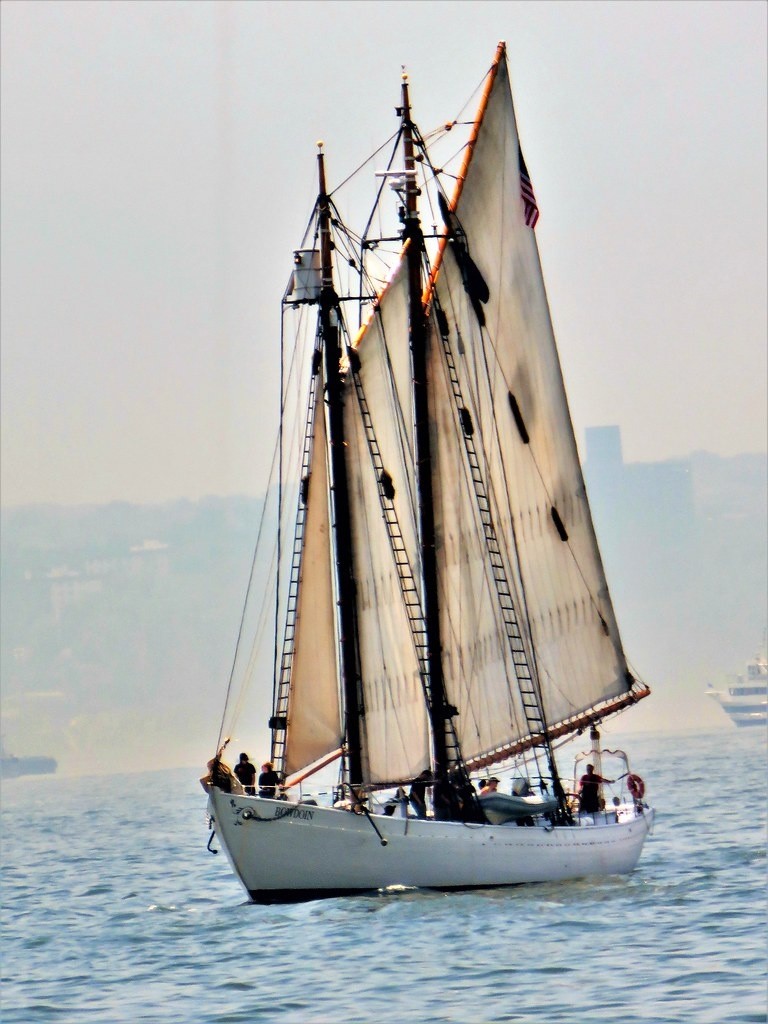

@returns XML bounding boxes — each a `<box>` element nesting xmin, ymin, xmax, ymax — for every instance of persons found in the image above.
<box><xmin>257</xmin><ymin>763</ymin><xmax>283</xmax><ymax>800</ymax></box>
<box><xmin>408</xmin><ymin>770</ymin><xmax>433</xmax><ymax>820</ymax></box>
<box><xmin>573</xmin><ymin>763</ymin><xmax>617</xmax><ymax>814</ymax></box>
<box><xmin>233</xmin><ymin>753</ymin><xmax>257</xmax><ymax>796</ymax></box>
<box><xmin>478</xmin><ymin>778</ymin><xmax>536</xmax><ymax>827</ymax></box>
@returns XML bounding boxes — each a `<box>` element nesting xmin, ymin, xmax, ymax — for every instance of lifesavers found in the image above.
<box><xmin>628</xmin><ymin>775</ymin><xmax>646</xmax><ymax>799</ymax></box>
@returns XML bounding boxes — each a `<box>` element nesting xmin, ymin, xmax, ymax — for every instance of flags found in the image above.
<box><xmin>519</xmin><ymin>147</ymin><xmax>539</xmax><ymax>229</ymax></box>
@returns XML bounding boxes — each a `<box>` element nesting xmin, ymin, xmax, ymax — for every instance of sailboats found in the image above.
<box><xmin>200</xmin><ymin>38</ymin><xmax>660</xmax><ymax>909</ymax></box>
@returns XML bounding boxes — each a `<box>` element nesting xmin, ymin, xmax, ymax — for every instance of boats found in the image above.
<box><xmin>706</xmin><ymin>652</ymin><xmax>768</xmax><ymax>731</ymax></box>
<box><xmin>0</xmin><ymin>734</ymin><xmax>63</xmax><ymax>778</ymax></box>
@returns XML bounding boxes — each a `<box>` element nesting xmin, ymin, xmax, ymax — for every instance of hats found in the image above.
<box><xmin>489</xmin><ymin>777</ymin><xmax>500</xmax><ymax>783</ymax></box>
<box><xmin>239</xmin><ymin>753</ymin><xmax>249</xmax><ymax>762</ymax></box>
<box><xmin>263</xmin><ymin>762</ymin><xmax>273</xmax><ymax>768</ymax></box>
